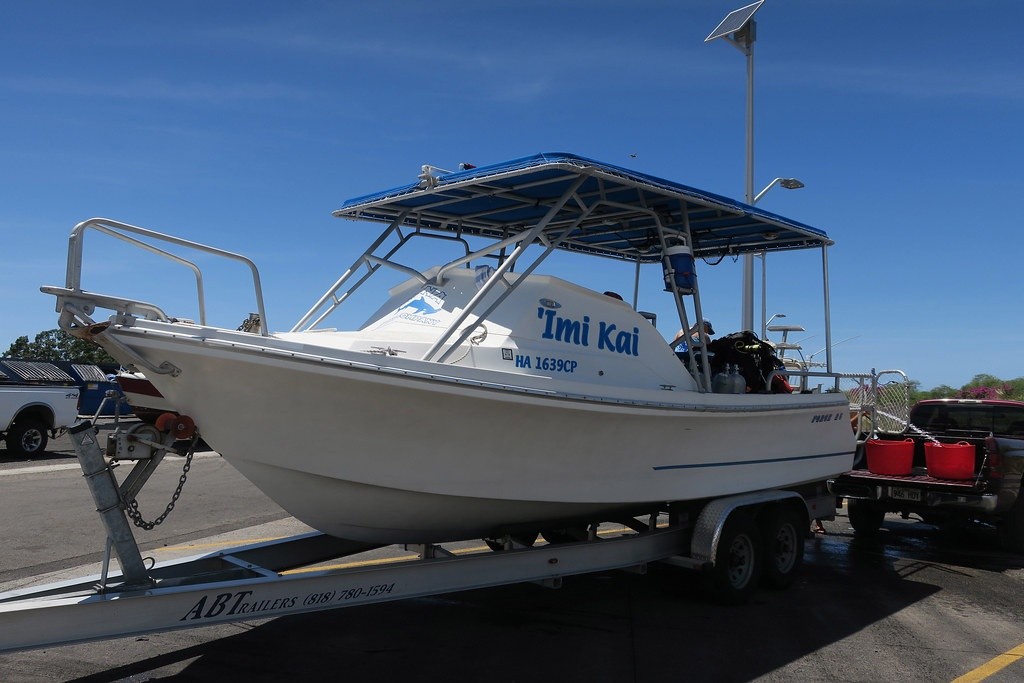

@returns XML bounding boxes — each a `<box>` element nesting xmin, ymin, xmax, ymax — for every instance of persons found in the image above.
<box><xmin>673</xmin><ymin>316</ymin><xmax>716</xmax><ymax>352</ymax></box>
<box><xmin>813</xmin><ymin>410</ymin><xmax>868</xmax><ymax>534</ymax></box>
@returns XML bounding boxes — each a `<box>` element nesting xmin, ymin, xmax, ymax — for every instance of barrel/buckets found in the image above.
<box><xmin>865</xmin><ymin>438</ymin><xmax>915</xmax><ymax>475</ymax></box>
<box><xmin>923</xmin><ymin>440</ymin><xmax>975</xmax><ymax>480</ymax></box>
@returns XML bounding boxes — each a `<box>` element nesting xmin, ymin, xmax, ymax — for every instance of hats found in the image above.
<box><xmin>702</xmin><ymin>317</ymin><xmax>715</xmax><ymax>335</ymax></box>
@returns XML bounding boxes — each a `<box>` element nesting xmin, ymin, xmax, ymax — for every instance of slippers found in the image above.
<box><xmin>814</xmin><ymin>528</ymin><xmax>826</xmax><ymax>534</ymax></box>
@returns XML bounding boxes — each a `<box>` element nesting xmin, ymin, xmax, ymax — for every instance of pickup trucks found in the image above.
<box><xmin>826</xmin><ymin>398</ymin><xmax>1024</xmax><ymax>532</ymax></box>
<box><xmin>0</xmin><ymin>386</ymin><xmax>81</xmax><ymax>460</ymax></box>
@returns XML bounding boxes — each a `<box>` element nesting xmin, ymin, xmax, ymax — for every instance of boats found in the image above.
<box><xmin>39</xmin><ymin>151</ymin><xmax>859</xmax><ymax>547</ymax></box>
<box><xmin>106</xmin><ymin>370</ymin><xmax>201</xmax><ymax>437</ymax></box>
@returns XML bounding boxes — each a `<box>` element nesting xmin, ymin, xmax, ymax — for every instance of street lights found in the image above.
<box><xmin>705</xmin><ymin>0</ymin><xmax>805</xmax><ymax>337</ymax></box>
